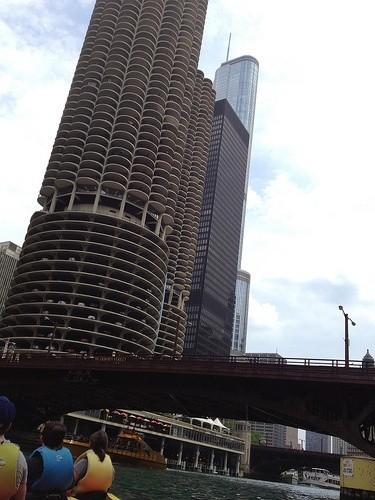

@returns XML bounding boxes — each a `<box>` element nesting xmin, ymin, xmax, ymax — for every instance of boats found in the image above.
<box><xmin>33</xmin><ymin>425</ymin><xmax>166</xmax><ymax>469</ymax></box>
<box><xmin>302</xmin><ymin>468</ymin><xmax>340</xmax><ymax>490</ymax></box>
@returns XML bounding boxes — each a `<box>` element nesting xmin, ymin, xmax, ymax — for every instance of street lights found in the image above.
<box><xmin>45</xmin><ymin>316</ymin><xmax>71</xmax><ymax>353</ymax></box>
<box><xmin>0</xmin><ymin>337</ymin><xmax>16</xmax><ymax>358</ymax></box>
<box><xmin>168</xmin><ymin>311</ymin><xmax>193</xmax><ymax>360</ymax></box>
<box><xmin>338</xmin><ymin>305</ymin><xmax>357</xmax><ymax>367</ymax></box>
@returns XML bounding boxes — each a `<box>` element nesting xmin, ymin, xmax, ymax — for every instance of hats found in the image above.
<box><xmin>0</xmin><ymin>396</ymin><xmax>16</xmax><ymax>421</ymax></box>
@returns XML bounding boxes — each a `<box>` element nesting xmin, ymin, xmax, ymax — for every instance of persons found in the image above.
<box><xmin>36</xmin><ymin>419</ymin><xmax>51</xmax><ymax>439</ymax></box>
<box><xmin>25</xmin><ymin>420</ymin><xmax>75</xmax><ymax>500</ymax></box>
<box><xmin>107</xmin><ymin>426</ymin><xmax>142</xmax><ymax>453</ymax></box>
<box><xmin>66</xmin><ymin>429</ymin><xmax>115</xmax><ymax>498</ymax></box>
<box><xmin>0</xmin><ymin>396</ymin><xmax>28</xmax><ymax>500</ymax></box>
